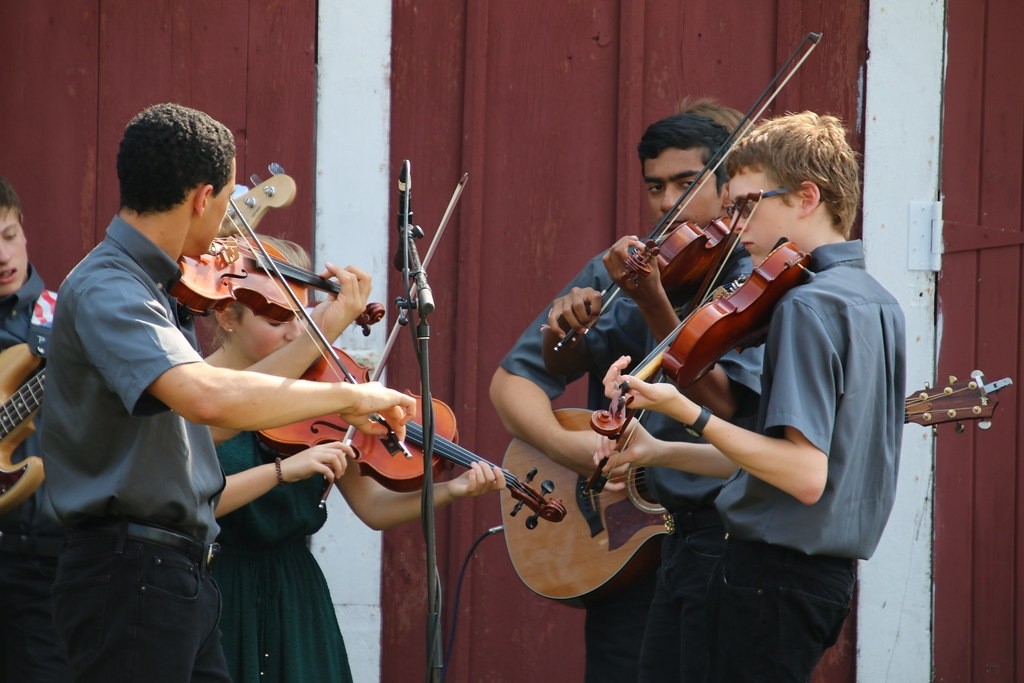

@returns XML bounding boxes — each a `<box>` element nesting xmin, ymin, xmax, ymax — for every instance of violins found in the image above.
<box><xmin>166</xmin><ymin>234</ymin><xmax>387</xmax><ymax>338</ymax></box>
<box><xmin>250</xmin><ymin>344</ymin><xmax>568</xmax><ymax>531</ymax></box>
<box><xmin>590</xmin><ymin>242</ymin><xmax>820</xmax><ymax>440</ymax></box>
<box><xmin>610</xmin><ymin>211</ymin><xmax>741</xmax><ymax>299</ymax></box>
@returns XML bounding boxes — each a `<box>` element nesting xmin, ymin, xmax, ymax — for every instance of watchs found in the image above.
<box><xmin>684</xmin><ymin>404</ymin><xmax>714</xmax><ymax>439</ymax></box>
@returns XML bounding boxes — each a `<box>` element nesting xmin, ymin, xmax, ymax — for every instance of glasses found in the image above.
<box><xmin>726</xmin><ymin>184</ymin><xmax>825</xmax><ymax>220</ymax></box>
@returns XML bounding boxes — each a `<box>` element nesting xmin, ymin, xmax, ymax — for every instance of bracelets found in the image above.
<box><xmin>273</xmin><ymin>457</ymin><xmax>284</xmax><ymax>485</ymax></box>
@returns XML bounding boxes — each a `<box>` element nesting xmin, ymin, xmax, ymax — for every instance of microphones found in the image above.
<box><xmin>393</xmin><ymin>159</ymin><xmax>412</xmax><ymax>272</ymax></box>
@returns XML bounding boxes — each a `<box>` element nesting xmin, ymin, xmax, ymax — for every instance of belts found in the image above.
<box><xmin>0</xmin><ymin>532</ymin><xmax>63</xmax><ymax>557</ymax></box>
<box><xmin>74</xmin><ymin>517</ymin><xmax>221</xmax><ymax>568</ymax></box>
<box><xmin>662</xmin><ymin>507</ymin><xmax>723</xmax><ymax>535</ymax></box>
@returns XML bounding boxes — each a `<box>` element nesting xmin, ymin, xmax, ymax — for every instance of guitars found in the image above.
<box><xmin>0</xmin><ymin>162</ymin><xmax>296</xmax><ymax>518</ymax></box>
<box><xmin>495</xmin><ymin>370</ymin><xmax>1014</xmax><ymax>606</ymax></box>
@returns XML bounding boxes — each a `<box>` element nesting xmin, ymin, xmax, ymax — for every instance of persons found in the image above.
<box><xmin>490</xmin><ymin>95</ymin><xmax>906</xmax><ymax>683</ymax></box>
<box><xmin>0</xmin><ymin>102</ymin><xmax>507</xmax><ymax>683</ymax></box>
